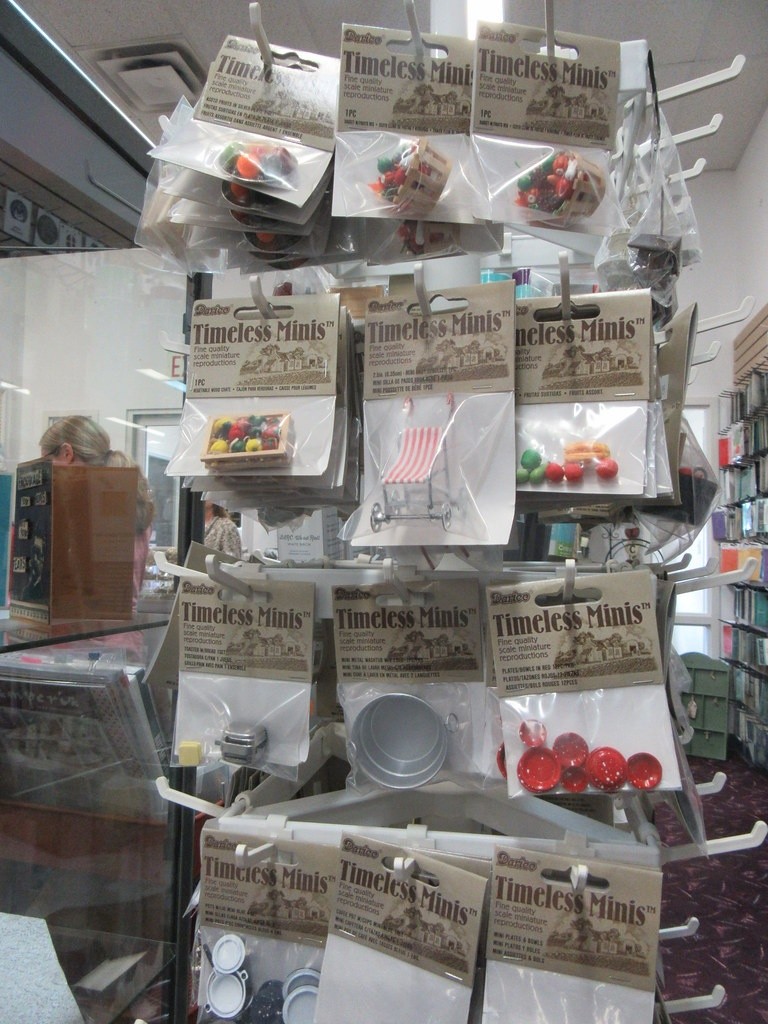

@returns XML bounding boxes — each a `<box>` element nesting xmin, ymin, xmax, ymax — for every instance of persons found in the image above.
<box><xmin>38</xmin><ymin>415</ymin><xmax>154</xmax><ymax>665</ymax></box>
<box><xmin>166</xmin><ymin>488</ymin><xmax>242</xmax><ymax>565</ymax></box>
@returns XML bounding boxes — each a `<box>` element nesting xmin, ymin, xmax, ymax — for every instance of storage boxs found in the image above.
<box><xmin>0</xmin><ymin>800</ymin><xmax>223</xmax><ymax>884</ymax></box>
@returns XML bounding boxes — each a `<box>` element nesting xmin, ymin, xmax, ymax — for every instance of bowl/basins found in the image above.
<box><xmin>347</xmin><ymin>692</ymin><xmax>450</xmax><ymax>789</ymax></box>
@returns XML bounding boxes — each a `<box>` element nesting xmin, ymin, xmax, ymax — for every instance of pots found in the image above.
<box><xmin>200</xmin><ymin>944</ymin><xmax>250</xmax><ymax>1018</ymax></box>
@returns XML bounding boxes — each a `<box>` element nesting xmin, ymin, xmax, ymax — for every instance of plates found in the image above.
<box><xmin>204</xmin><ymin>934</ymin><xmax>321</xmax><ymax>1024</ymax></box>
<box><xmin>517</xmin><ymin>719</ymin><xmax>664</xmax><ymax>792</ymax></box>
